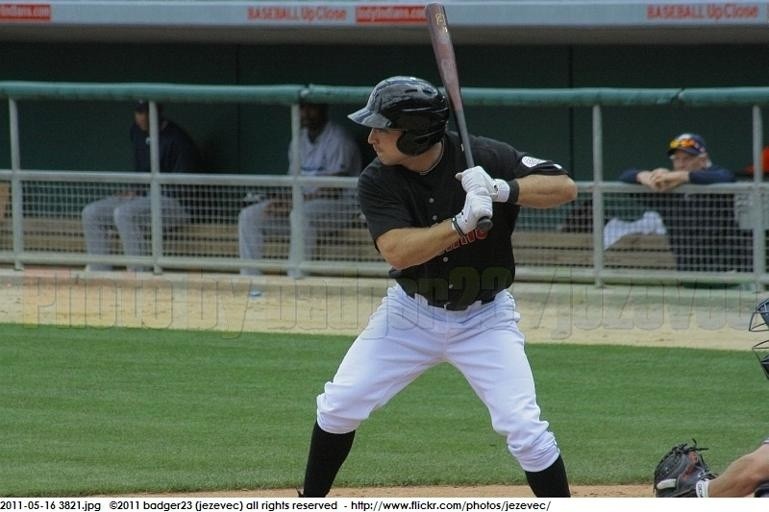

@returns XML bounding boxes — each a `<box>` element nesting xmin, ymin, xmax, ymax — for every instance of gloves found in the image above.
<box><xmin>450</xmin><ymin>187</ymin><xmax>493</xmax><ymax>238</ymax></box>
<box><xmin>454</xmin><ymin>165</ymin><xmax>510</xmax><ymax>203</ymax></box>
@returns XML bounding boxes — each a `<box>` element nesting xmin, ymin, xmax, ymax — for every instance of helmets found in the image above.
<box><xmin>749</xmin><ymin>297</ymin><xmax>769</xmax><ymax>382</ymax></box>
<box><xmin>347</xmin><ymin>75</ymin><xmax>451</xmax><ymax>156</ymax></box>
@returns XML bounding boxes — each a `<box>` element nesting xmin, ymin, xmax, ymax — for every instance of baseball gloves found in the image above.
<box><xmin>653</xmin><ymin>445</ymin><xmax>715</xmax><ymax>497</ymax></box>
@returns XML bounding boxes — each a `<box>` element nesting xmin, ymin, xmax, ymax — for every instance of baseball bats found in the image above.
<box><xmin>424</xmin><ymin>3</ymin><xmax>493</xmax><ymax>232</ymax></box>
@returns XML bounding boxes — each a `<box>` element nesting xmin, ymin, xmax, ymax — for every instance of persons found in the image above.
<box><xmin>237</xmin><ymin>101</ymin><xmax>364</xmax><ymax>298</ymax></box>
<box><xmin>79</xmin><ymin>102</ymin><xmax>201</xmax><ymax>271</ymax></box>
<box><xmin>619</xmin><ymin>132</ymin><xmax>745</xmax><ymax>290</ymax></box>
<box><xmin>653</xmin><ymin>298</ymin><xmax>769</xmax><ymax>497</ymax></box>
<box><xmin>294</xmin><ymin>74</ymin><xmax>579</xmax><ymax>498</ymax></box>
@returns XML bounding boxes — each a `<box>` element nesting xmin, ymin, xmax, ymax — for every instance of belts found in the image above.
<box><xmin>410</xmin><ymin>293</ymin><xmax>495</xmax><ymax>312</ymax></box>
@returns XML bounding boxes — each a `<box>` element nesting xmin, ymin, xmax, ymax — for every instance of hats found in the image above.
<box><xmin>132</xmin><ymin>101</ymin><xmax>149</xmax><ymax>112</ymax></box>
<box><xmin>665</xmin><ymin>133</ymin><xmax>708</xmax><ymax>156</ymax></box>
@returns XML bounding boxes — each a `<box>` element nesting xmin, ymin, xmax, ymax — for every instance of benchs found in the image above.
<box><xmin>0</xmin><ymin>207</ymin><xmax>680</xmax><ymax>285</ymax></box>
